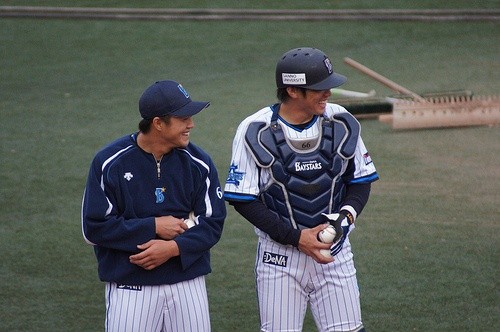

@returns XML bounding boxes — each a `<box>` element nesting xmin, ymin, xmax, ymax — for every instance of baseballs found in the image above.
<box><xmin>319</xmin><ymin>249</ymin><xmax>333</xmax><ymax>259</ymax></box>
<box><xmin>318</xmin><ymin>225</ymin><xmax>336</xmax><ymax>243</ymax></box>
<box><xmin>182</xmin><ymin>219</ymin><xmax>195</xmax><ymax>228</ymax></box>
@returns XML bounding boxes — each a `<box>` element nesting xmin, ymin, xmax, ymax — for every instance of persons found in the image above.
<box><xmin>222</xmin><ymin>47</ymin><xmax>380</xmax><ymax>332</ymax></box>
<box><xmin>80</xmin><ymin>81</ymin><xmax>227</xmax><ymax>332</ymax></box>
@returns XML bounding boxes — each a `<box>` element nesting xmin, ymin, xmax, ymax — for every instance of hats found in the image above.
<box><xmin>139</xmin><ymin>79</ymin><xmax>210</xmax><ymax>119</ymax></box>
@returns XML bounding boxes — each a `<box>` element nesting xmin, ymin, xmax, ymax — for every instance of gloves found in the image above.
<box><xmin>321</xmin><ymin>211</ymin><xmax>352</xmax><ymax>256</ymax></box>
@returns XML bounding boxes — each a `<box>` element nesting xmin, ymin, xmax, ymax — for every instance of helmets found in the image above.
<box><xmin>275</xmin><ymin>47</ymin><xmax>347</xmax><ymax>91</ymax></box>
